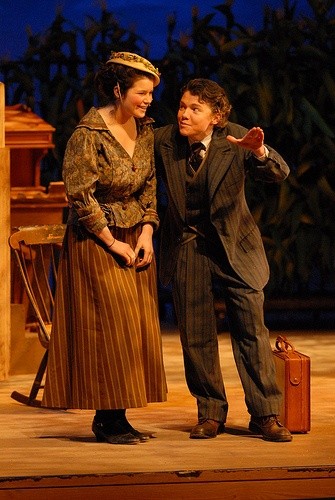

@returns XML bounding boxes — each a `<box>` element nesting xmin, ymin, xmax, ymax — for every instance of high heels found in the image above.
<box><xmin>133</xmin><ymin>430</ymin><xmax>149</xmax><ymax>442</ymax></box>
<box><xmin>91</xmin><ymin>423</ymin><xmax>142</xmax><ymax>445</ymax></box>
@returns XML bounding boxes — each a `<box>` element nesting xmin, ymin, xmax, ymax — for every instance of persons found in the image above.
<box><xmin>42</xmin><ymin>52</ymin><xmax>168</xmax><ymax>445</ymax></box>
<box><xmin>153</xmin><ymin>78</ymin><xmax>293</xmax><ymax>442</ymax></box>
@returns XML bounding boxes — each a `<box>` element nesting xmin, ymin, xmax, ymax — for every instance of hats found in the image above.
<box><xmin>106</xmin><ymin>52</ymin><xmax>160</xmax><ymax>87</ymax></box>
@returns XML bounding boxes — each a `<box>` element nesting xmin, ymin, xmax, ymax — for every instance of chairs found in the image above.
<box><xmin>7</xmin><ymin>224</ymin><xmax>68</xmax><ymax>411</ymax></box>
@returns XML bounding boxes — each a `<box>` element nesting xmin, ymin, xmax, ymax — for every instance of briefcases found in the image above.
<box><xmin>272</xmin><ymin>336</ymin><xmax>311</xmax><ymax>433</ymax></box>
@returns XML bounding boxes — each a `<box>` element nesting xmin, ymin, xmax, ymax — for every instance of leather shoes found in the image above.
<box><xmin>190</xmin><ymin>419</ymin><xmax>224</xmax><ymax>439</ymax></box>
<box><xmin>249</xmin><ymin>416</ymin><xmax>291</xmax><ymax>442</ymax></box>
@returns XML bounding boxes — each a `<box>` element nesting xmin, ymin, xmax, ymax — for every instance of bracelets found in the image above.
<box><xmin>107</xmin><ymin>237</ymin><xmax>116</xmax><ymax>248</ymax></box>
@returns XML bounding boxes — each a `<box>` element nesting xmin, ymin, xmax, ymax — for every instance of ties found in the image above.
<box><xmin>191</xmin><ymin>143</ymin><xmax>206</xmax><ymax>171</ymax></box>
